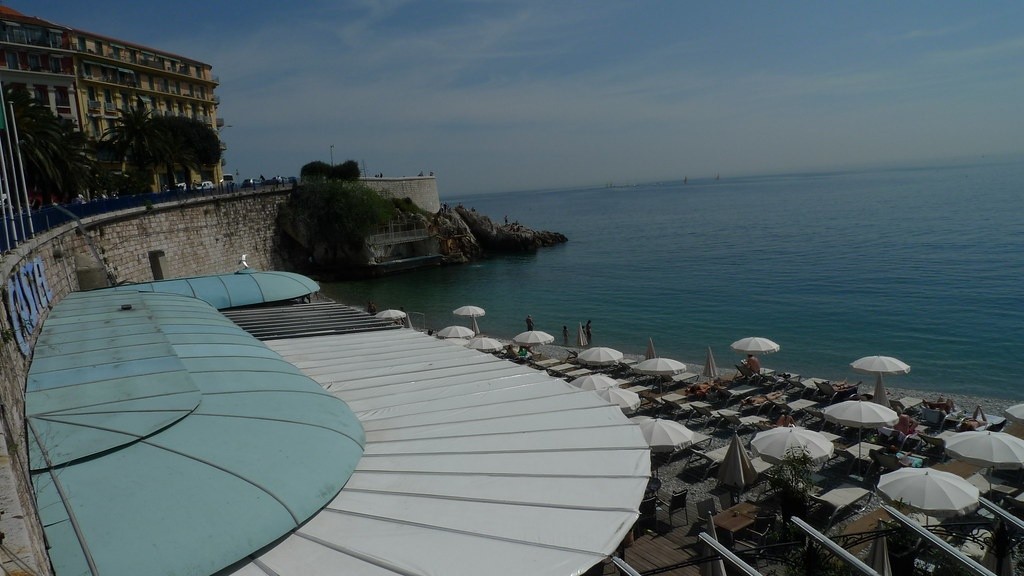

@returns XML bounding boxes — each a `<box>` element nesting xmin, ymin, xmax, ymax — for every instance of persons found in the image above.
<box><xmin>891</xmin><ymin>406</ymin><xmax>918</xmax><ymax>434</ymax></box>
<box><xmin>508</xmin><ymin>343</ymin><xmax>527</xmax><ymax>357</ymax></box>
<box><xmin>687</xmin><ymin>379</ymin><xmax>719</xmax><ymax>392</ymax></box>
<box><xmin>849</xmin><ymin>390</ymin><xmax>875</xmax><ymax>402</ymax></box>
<box><xmin>923</xmin><ymin>395</ymin><xmax>957</xmax><ymax>416</ymax></box>
<box><xmin>525</xmin><ymin>314</ymin><xmax>535</xmax><ymax>331</ymax></box>
<box><xmin>561</xmin><ymin>324</ymin><xmax>571</xmax><ymax>345</ymax></box>
<box><xmin>777</xmin><ymin>409</ymin><xmax>796</xmax><ymax>428</ymax></box>
<box><xmin>955</xmin><ymin>404</ymin><xmax>988</xmax><ymax>430</ymax></box>
<box><xmin>822</xmin><ymin>376</ymin><xmax>862</xmax><ymax>390</ymax></box>
<box><xmin>744</xmin><ymin>390</ymin><xmax>782</xmax><ymax>404</ymax></box>
<box><xmin>743</xmin><ymin>354</ymin><xmax>760</xmax><ymax>376</ymax></box>
<box><xmin>586</xmin><ymin>319</ymin><xmax>592</xmax><ymax>343</ymax></box>
<box><xmin>581</xmin><ymin>325</ymin><xmax>586</xmax><ymax>335</ymax></box>
<box><xmin>0</xmin><ymin>169</ymin><xmax>520</xmax><ymax>232</ymax></box>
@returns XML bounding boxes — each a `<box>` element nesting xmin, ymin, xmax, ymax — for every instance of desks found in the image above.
<box><xmin>713</xmin><ymin>500</ymin><xmax>766</xmax><ymax>544</ymax></box>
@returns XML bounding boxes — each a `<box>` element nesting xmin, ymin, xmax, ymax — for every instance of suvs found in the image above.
<box><xmin>243</xmin><ymin>179</ymin><xmax>262</xmax><ymax>186</ymax></box>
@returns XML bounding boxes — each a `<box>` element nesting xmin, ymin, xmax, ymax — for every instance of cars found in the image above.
<box><xmin>265</xmin><ymin>176</ymin><xmax>297</xmax><ymax>184</ymax></box>
<box><xmin>72</xmin><ymin>194</ymin><xmax>117</xmax><ymax>205</ymax></box>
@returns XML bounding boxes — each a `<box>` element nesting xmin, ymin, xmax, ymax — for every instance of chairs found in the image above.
<box><xmin>483</xmin><ymin>342</ymin><xmax>1024</xmax><ymax>576</ymax></box>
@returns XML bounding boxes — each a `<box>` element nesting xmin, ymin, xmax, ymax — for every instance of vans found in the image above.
<box><xmin>194</xmin><ymin>181</ymin><xmax>213</xmax><ymax>190</ymax></box>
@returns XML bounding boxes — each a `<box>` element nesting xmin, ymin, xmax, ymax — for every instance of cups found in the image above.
<box><xmin>734</xmin><ymin>510</ymin><xmax>739</xmax><ymax>517</ymax></box>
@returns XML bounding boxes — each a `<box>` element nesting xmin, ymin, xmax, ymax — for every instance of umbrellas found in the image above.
<box><xmin>371</xmin><ymin>305</ymin><xmax>1024</xmax><ymax>533</ymax></box>
